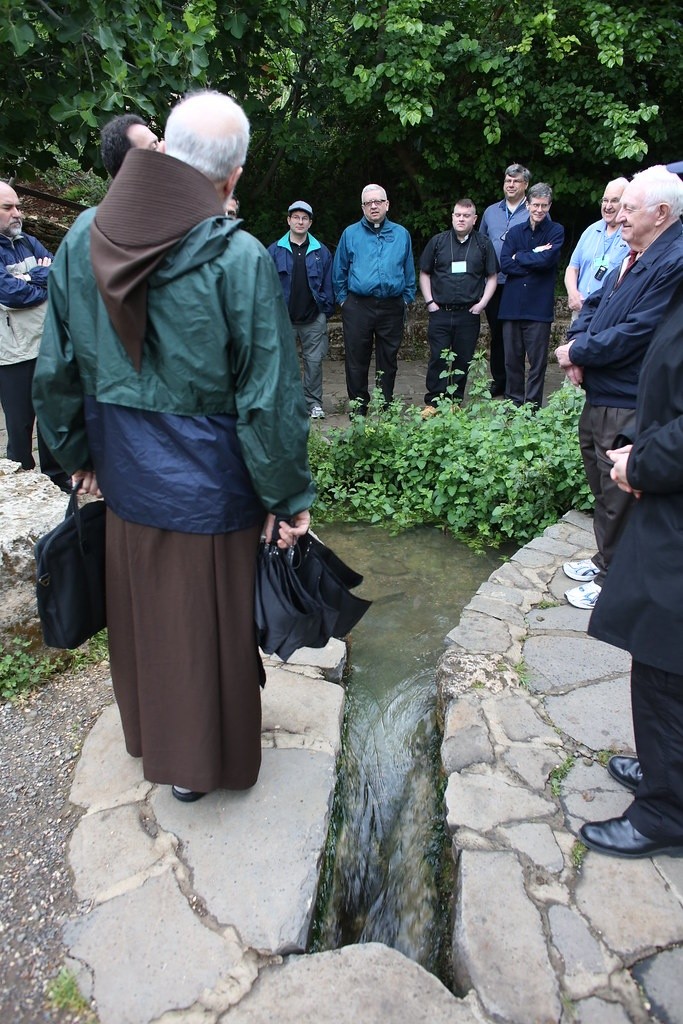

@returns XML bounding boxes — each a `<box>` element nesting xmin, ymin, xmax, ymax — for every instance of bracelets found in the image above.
<box><xmin>426</xmin><ymin>300</ymin><xmax>434</xmax><ymax>306</ymax></box>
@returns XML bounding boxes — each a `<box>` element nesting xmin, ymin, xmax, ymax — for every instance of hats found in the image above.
<box><xmin>667</xmin><ymin>160</ymin><xmax>683</xmax><ymax>173</ymax></box>
<box><xmin>288</xmin><ymin>200</ymin><xmax>312</xmax><ymax>214</ymax></box>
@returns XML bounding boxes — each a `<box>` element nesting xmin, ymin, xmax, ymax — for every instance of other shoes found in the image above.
<box><xmin>449</xmin><ymin>405</ymin><xmax>461</xmax><ymax>413</ymax></box>
<box><xmin>172</xmin><ymin>784</ymin><xmax>206</xmax><ymax>801</ymax></box>
<box><xmin>52</xmin><ymin>472</ymin><xmax>72</xmax><ymax>494</ymax></box>
<box><xmin>420</xmin><ymin>405</ymin><xmax>436</xmax><ymax>420</ymax></box>
<box><xmin>349</xmin><ymin>409</ymin><xmax>367</xmax><ymax>420</ymax></box>
<box><xmin>379</xmin><ymin>404</ymin><xmax>388</xmax><ymax>412</ymax></box>
<box><xmin>488</xmin><ymin>382</ymin><xmax>502</xmax><ymax>398</ymax></box>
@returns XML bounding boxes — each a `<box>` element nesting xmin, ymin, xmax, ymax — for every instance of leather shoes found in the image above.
<box><xmin>578</xmin><ymin>816</ymin><xmax>683</xmax><ymax>857</ymax></box>
<box><xmin>606</xmin><ymin>754</ymin><xmax>644</xmax><ymax>790</ymax></box>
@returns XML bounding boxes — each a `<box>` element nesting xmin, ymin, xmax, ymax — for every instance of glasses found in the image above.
<box><xmin>503</xmin><ymin>179</ymin><xmax>525</xmax><ymax>184</ymax></box>
<box><xmin>362</xmin><ymin>199</ymin><xmax>386</xmax><ymax>207</ymax></box>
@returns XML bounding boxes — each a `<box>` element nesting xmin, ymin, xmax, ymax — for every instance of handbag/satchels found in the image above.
<box><xmin>33</xmin><ymin>476</ymin><xmax>107</xmax><ymax>650</ymax></box>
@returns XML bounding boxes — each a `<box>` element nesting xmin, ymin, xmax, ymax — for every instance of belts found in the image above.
<box><xmin>437</xmin><ymin>300</ymin><xmax>479</xmax><ymax>311</ymax></box>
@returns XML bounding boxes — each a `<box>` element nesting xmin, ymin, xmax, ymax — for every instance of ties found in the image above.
<box><xmin>627</xmin><ymin>251</ymin><xmax>638</xmax><ymax>269</ymax></box>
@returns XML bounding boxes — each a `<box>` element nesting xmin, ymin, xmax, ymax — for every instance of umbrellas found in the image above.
<box><xmin>254</xmin><ymin>516</ymin><xmax>372</xmax><ymax>661</ymax></box>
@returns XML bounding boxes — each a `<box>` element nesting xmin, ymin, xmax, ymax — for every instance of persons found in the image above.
<box><xmin>32</xmin><ymin>91</ymin><xmax>312</xmax><ymax>802</ymax></box>
<box><xmin>100</xmin><ymin>113</ymin><xmax>160</xmax><ymax>179</ymax></box>
<box><xmin>0</xmin><ymin>180</ymin><xmax>73</xmax><ymax>494</ymax></box>
<box><xmin>477</xmin><ymin>163</ymin><xmax>553</xmax><ymax>399</ymax></box>
<box><xmin>496</xmin><ymin>183</ymin><xmax>564</xmax><ymax>413</ymax></box>
<box><xmin>564</xmin><ymin>176</ymin><xmax>630</xmax><ymax>328</ymax></box>
<box><xmin>227</xmin><ymin>195</ymin><xmax>239</xmax><ymax>218</ymax></box>
<box><xmin>416</xmin><ymin>198</ymin><xmax>502</xmax><ymax>419</ymax></box>
<box><xmin>265</xmin><ymin>200</ymin><xmax>332</xmax><ymax>419</ymax></box>
<box><xmin>580</xmin><ymin>281</ymin><xmax>683</xmax><ymax>860</ymax></box>
<box><xmin>555</xmin><ymin>164</ymin><xmax>683</xmax><ymax>611</ymax></box>
<box><xmin>332</xmin><ymin>184</ymin><xmax>415</xmax><ymax>421</ymax></box>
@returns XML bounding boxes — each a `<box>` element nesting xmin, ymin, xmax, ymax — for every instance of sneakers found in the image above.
<box><xmin>564</xmin><ymin>580</ymin><xmax>601</xmax><ymax>610</ymax></box>
<box><xmin>562</xmin><ymin>558</ymin><xmax>601</xmax><ymax>581</ymax></box>
<box><xmin>310</xmin><ymin>406</ymin><xmax>325</xmax><ymax>418</ymax></box>
